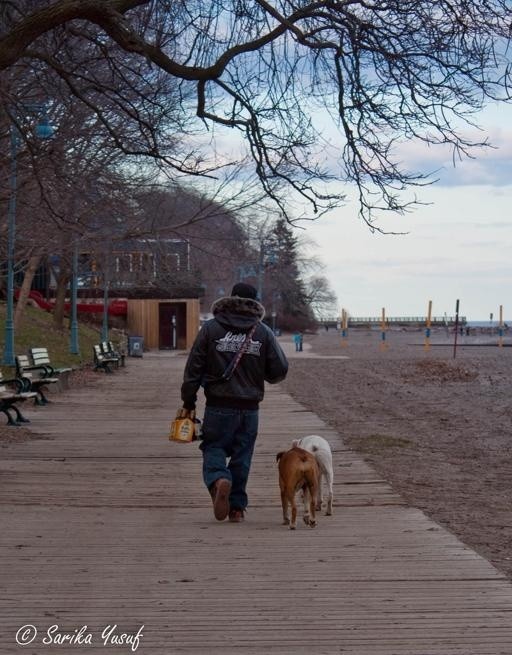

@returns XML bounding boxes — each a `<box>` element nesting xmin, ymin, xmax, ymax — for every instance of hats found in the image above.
<box><xmin>232</xmin><ymin>282</ymin><xmax>259</xmax><ymax>299</ymax></box>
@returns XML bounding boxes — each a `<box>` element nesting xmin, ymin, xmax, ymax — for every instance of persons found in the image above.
<box><xmin>175</xmin><ymin>280</ymin><xmax>290</xmax><ymax>524</ymax></box>
<box><xmin>292</xmin><ymin>331</ymin><xmax>300</xmax><ymax>352</ymax></box>
<box><xmin>297</xmin><ymin>330</ymin><xmax>303</xmax><ymax>352</ymax></box>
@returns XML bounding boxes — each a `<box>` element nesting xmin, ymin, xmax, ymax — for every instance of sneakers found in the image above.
<box><xmin>229</xmin><ymin>509</ymin><xmax>246</xmax><ymax>522</ymax></box>
<box><xmin>209</xmin><ymin>477</ymin><xmax>230</xmax><ymax>520</ymax></box>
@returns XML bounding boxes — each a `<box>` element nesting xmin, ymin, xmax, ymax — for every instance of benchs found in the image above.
<box><xmin>92</xmin><ymin>341</ymin><xmax>126</xmax><ymax>373</ymax></box>
<box><xmin>0</xmin><ymin>347</ymin><xmax>74</xmax><ymax>425</ymax></box>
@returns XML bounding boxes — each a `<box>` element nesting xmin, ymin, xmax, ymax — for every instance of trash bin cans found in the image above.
<box><xmin>129</xmin><ymin>336</ymin><xmax>144</xmax><ymax>358</ymax></box>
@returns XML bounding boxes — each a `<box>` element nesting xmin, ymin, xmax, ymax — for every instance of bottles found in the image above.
<box><xmin>168</xmin><ymin>408</ymin><xmax>202</xmax><ymax>443</ymax></box>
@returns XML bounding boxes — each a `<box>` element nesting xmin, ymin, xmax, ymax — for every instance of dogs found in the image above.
<box><xmin>276</xmin><ymin>447</ymin><xmax>317</xmax><ymax>531</ymax></box>
<box><xmin>292</xmin><ymin>435</ymin><xmax>333</xmax><ymax>517</ymax></box>
